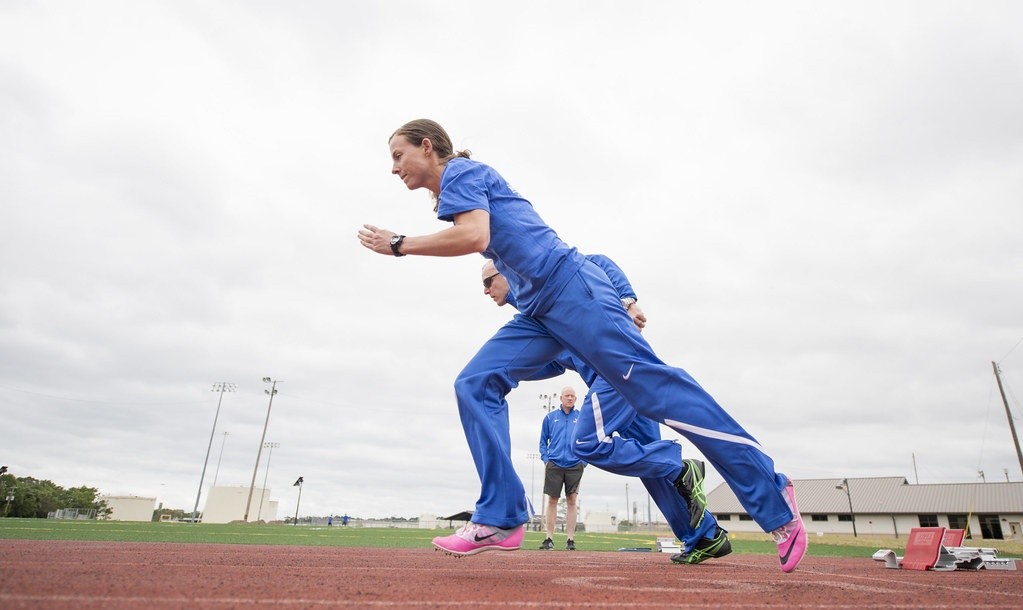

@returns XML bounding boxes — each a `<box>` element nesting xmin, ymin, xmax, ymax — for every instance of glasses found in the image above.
<box><xmin>483</xmin><ymin>273</ymin><xmax>501</xmax><ymax>287</ymax></box>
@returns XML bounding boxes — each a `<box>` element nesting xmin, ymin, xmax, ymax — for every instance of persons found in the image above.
<box><xmin>481</xmin><ymin>255</ymin><xmax>732</xmax><ymax>563</ymax></box>
<box><xmin>328</xmin><ymin>514</ymin><xmax>333</xmax><ymax>526</ymax></box>
<box><xmin>342</xmin><ymin>514</ymin><xmax>348</xmax><ymax>525</ymax></box>
<box><xmin>540</xmin><ymin>388</ymin><xmax>586</xmax><ymax>552</ymax></box>
<box><xmin>359</xmin><ymin>120</ymin><xmax>809</xmax><ymax>573</ymax></box>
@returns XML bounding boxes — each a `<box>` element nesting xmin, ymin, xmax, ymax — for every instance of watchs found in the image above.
<box><xmin>390</xmin><ymin>234</ymin><xmax>406</xmax><ymax>257</ymax></box>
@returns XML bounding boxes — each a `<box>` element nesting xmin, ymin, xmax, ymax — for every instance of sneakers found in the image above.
<box><xmin>565</xmin><ymin>539</ymin><xmax>573</xmax><ymax>549</ymax></box>
<box><xmin>539</xmin><ymin>537</ymin><xmax>554</xmax><ymax>550</ymax></box>
<box><xmin>771</xmin><ymin>476</ymin><xmax>810</xmax><ymax>571</ymax></box>
<box><xmin>671</xmin><ymin>528</ymin><xmax>732</xmax><ymax>564</ymax></box>
<box><xmin>432</xmin><ymin>522</ymin><xmax>524</xmax><ymax>557</ymax></box>
<box><xmin>673</xmin><ymin>458</ymin><xmax>706</xmax><ymax>528</ymax></box>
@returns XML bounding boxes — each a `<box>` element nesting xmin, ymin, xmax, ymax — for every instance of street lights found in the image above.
<box><xmin>254</xmin><ymin>441</ymin><xmax>279</xmax><ymax>521</ymax></box>
<box><xmin>243</xmin><ymin>376</ymin><xmax>279</xmax><ymax>520</ymax></box>
<box><xmin>191</xmin><ymin>381</ymin><xmax>237</xmax><ymax>526</ymax></box>
<box><xmin>525</xmin><ymin>453</ymin><xmax>541</xmax><ymax>518</ymax></box>
<box><xmin>538</xmin><ymin>392</ymin><xmax>558</xmax><ymax>415</ymax></box>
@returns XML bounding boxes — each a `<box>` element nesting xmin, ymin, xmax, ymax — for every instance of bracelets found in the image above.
<box><xmin>623</xmin><ymin>297</ymin><xmax>635</xmax><ymax>309</ymax></box>
